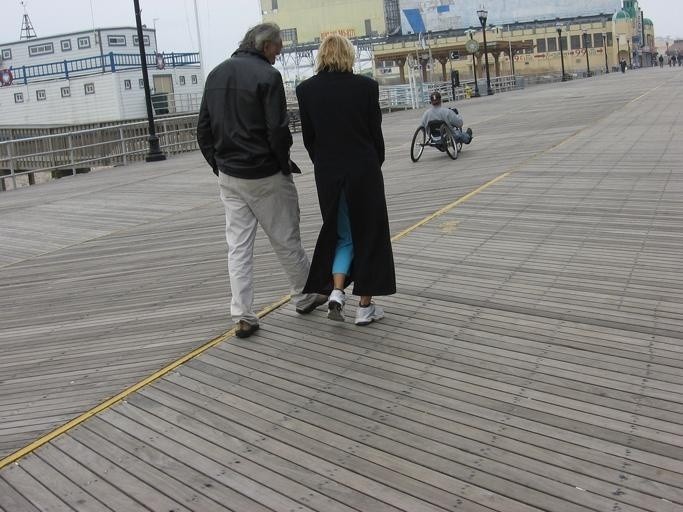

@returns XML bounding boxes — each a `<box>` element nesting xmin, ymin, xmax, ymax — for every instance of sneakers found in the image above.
<box><xmin>294</xmin><ymin>292</ymin><xmax>328</xmax><ymax>315</ymax></box>
<box><xmin>353</xmin><ymin>301</ymin><xmax>386</xmax><ymax>326</ymax></box>
<box><xmin>464</xmin><ymin>128</ymin><xmax>472</xmax><ymax>144</ymax></box>
<box><xmin>326</xmin><ymin>288</ymin><xmax>346</xmax><ymax>322</ymax></box>
<box><xmin>234</xmin><ymin>320</ymin><xmax>260</xmax><ymax>338</ymax></box>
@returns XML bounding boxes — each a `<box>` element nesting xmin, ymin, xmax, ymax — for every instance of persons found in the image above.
<box><xmin>294</xmin><ymin>33</ymin><xmax>397</xmax><ymax>325</ymax></box>
<box><xmin>620</xmin><ymin>58</ymin><xmax>627</xmax><ymax>73</ymax></box>
<box><xmin>651</xmin><ymin>52</ymin><xmax>683</xmax><ymax>68</ymax></box>
<box><xmin>422</xmin><ymin>91</ymin><xmax>472</xmax><ymax>144</ymax></box>
<box><xmin>195</xmin><ymin>23</ymin><xmax>328</xmax><ymax>338</ymax></box>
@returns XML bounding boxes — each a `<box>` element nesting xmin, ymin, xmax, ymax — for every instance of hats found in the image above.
<box><xmin>430</xmin><ymin>92</ymin><xmax>441</xmax><ymax>105</ymax></box>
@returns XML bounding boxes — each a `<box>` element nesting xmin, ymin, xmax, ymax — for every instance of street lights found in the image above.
<box><xmin>625</xmin><ymin>36</ymin><xmax>632</xmax><ymax>69</ymax></box>
<box><xmin>602</xmin><ymin>32</ymin><xmax>608</xmax><ymax>68</ymax></box>
<box><xmin>556</xmin><ymin>23</ymin><xmax>565</xmax><ymax>77</ymax></box>
<box><xmin>477</xmin><ymin>11</ymin><xmax>491</xmax><ymax>89</ymax></box>
<box><xmin>616</xmin><ymin>35</ymin><xmax>620</xmax><ymax>65</ymax></box>
<box><xmin>666</xmin><ymin>41</ymin><xmax>669</xmax><ymax>55</ymax></box>
<box><xmin>581</xmin><ymin>29</ymin><xmax>590</xmax><ymax>73</ymax></box>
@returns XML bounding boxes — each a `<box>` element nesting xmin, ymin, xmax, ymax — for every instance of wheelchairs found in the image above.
<box><xmin>411</xmin><ymin>107</ymin><xmax>462</xmax><ymax>161</ymax></box>
